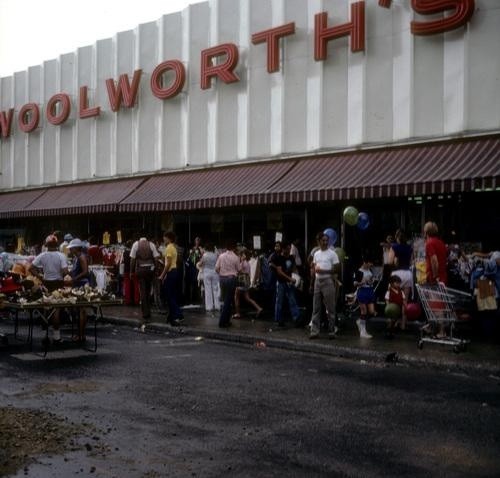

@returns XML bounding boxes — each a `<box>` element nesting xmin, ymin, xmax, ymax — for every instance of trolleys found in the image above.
<box><xmin>414</xmin><ymin>278</ymin><xmax>472</xmax><ymax>355</ymax></box>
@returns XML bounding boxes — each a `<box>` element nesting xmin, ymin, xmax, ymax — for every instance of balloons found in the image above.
<box><xmin>385</xmin><ymin>303</ymin><xmax>400</xmax><ymax>317</ymax></box>
<box><xmin>323</xmin><ymin>229</ymin><xmax>337</xmax><ymax>246</ymax></box>
<box><xmin>334</xmin><ymin>247</ymin><xmax>345</xmax><ymax>261</ymax></box>
<box><xmin>342</xmin><ymin>206</ymin><xmax>358</xmax><ymax>225</ymax></box>
<box><xmin>404</xmin><ymin>303</ymin><xmax>420</xmax><ymax>318</ymax></box>
<box><xmin>358</xmin><ymin>212</ymin><xmax>369</xmax><ymax>229</ymax></box>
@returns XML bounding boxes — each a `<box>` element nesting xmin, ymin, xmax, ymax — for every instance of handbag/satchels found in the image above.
<box><xmin>478</xmin><ymin>280</ymin><xmax>496</xmax><ymax>299</ymax></box>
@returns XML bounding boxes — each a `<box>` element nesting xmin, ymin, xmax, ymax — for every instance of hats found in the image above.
<box><xmin>66</xmin><ymin>238</ymin><xmax>86</xmax><ymax>249</ymax></box>
<box><xmin>64</xmin><ymin>234</ymin><xmax>73</xmax><ymax>241</ymax></box>
<box><xmin>45</xmin><ymin>235</ymin><xmax>58</xmax><ymax>247</ymax></box>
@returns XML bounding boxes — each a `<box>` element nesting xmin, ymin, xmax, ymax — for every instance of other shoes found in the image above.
<box><xmin>309</xmin><ymin>335</ymin><xmax>319</xmax><ymax>340</ymax></box>
<box><xmin>329</xmin><ymin>336</ymin><xmax>335</xmax><ymax>340</ymax></box>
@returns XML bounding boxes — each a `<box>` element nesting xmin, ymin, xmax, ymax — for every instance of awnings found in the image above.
<box><xmin>0</xmin><ymin>189</ymin><xmax>47</xmax><ymax>218</ymax></box>
<box><xmin>22</xmin><ymin>177</ymin><xmax>146</xmax><ymax>216</ymax></box>
<box><xmin>119</xmin><ymin>159</ymin><xmax>296</xmax><ymax>213</ymax></box>
<box><xmin>265</xmin><ymin>135</ymin><xmax>499</xmax><ymax>202</ymax></box>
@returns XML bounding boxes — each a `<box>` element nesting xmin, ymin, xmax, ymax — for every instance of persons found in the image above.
<box><xmin>157</xmin><ymin>232</ymin><xmax>184</xmax><ymax>325</ymax></box>
<box><xmin>120</xmin><ymin>240</ymin><xmax>140</xmax><ymax>305</ymax></box>
<box><xmin>102</xmin><ymin>252</ymin><xmax>116</xmax><ymax>266</ymax></box>
<box><xmin>215</xmin><ymin>241</ymin><xmax>242</xmax><ymax>328</ymax></box>
<box><xmin>185</xmin><ymin>235</ymin><xmax>304</xmax><ymax>327</ymax></box>
<box><xmin>424</xmin><ymin>222</ymin><xmax>448</xmax><ymax>339</ymax></box>
<box><xmin>306</xmin><ymin>227</ymin><xmax>500</xmax><ymax>341</ymax></box>
<box><xmin>129</xmin><ymin>231</ymin><xmax>159</xmax><ymax>324</ymax></box>
<box><xmin>0</xmin><ymin>231</ymin><xmax>104</xmax><ymax>341</ymax></box>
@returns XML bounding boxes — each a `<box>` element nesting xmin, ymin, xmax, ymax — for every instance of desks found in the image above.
<box><xmin>3</xmin><ymin>299</ymin><xmax>125</xmax><ymax>353</ymax></box>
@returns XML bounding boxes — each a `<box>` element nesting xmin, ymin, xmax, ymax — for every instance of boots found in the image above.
<box><xmin>356</xmin><ymin>319</ymin><xmax>360</xmax><ymax>332</ymax></box>
<box><xmin>360</xmin><ymin>319</ymin><xmax>373</xmax><ymax>338</ymax></box>
